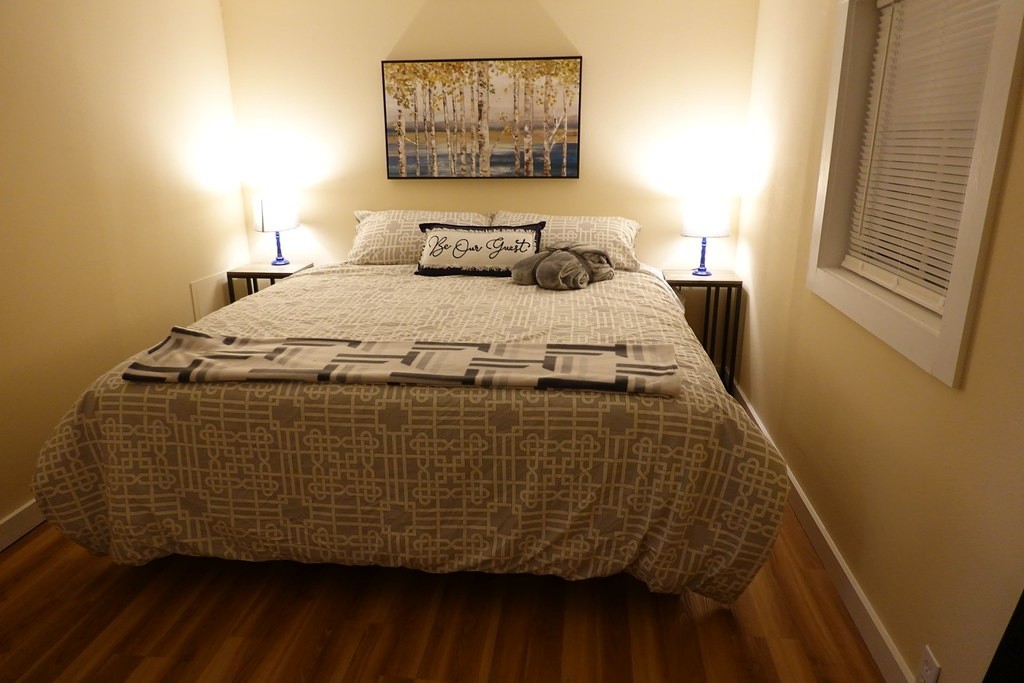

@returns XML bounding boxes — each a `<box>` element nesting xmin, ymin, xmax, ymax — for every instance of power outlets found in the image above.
<box><xmin>918</xmin><ymin>645</ymin><xmax>941</xmax><ymax>683</ymax></box>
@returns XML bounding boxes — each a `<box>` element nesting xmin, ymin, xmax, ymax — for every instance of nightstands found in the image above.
<box><xmin>663</xmin><ymin>267</ymin><xmax>743</xmax><ymax>397</ymax></box>
<box><xmin>226</xmin><ymin>262</ymin><xmax>313</xmax><ymax>304</ymax></box>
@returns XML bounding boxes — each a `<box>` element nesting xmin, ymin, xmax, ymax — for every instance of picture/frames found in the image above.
<box><xmin>381</xmin><ymin>56</ymin><xmax>583</xmax><ymax>180</ymax></box>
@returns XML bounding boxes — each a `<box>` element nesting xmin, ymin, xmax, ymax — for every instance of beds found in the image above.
<box><xmin>25</xmin><ymin>251</ymin><xmax>796</xmax><ymax>607</ymax></box>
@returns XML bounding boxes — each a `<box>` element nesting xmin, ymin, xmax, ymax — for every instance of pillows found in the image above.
<box><xmin>339</xmin><ymin>211</ymin><xmax>492</xmax><ymax>268</ymax></box>
<box><xmin>414</xmin><ymin>221</ymin><xmax>547</xmax><ymax>280</ymax></box>
<box><xmin>490</xmin><ymin>211</ymin><xmax>641</xmax><ymax>272</ymax></box>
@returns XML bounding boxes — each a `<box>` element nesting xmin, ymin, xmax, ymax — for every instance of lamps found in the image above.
<box><xmin>679</xmin><ymin>187</ymin><xmax>731</xmax><ymax>275</ymax></box>
<box><xmin>247</xmin><ymin>192</ymin><xmax>291</xmax><ymax>266</ymax></box>
